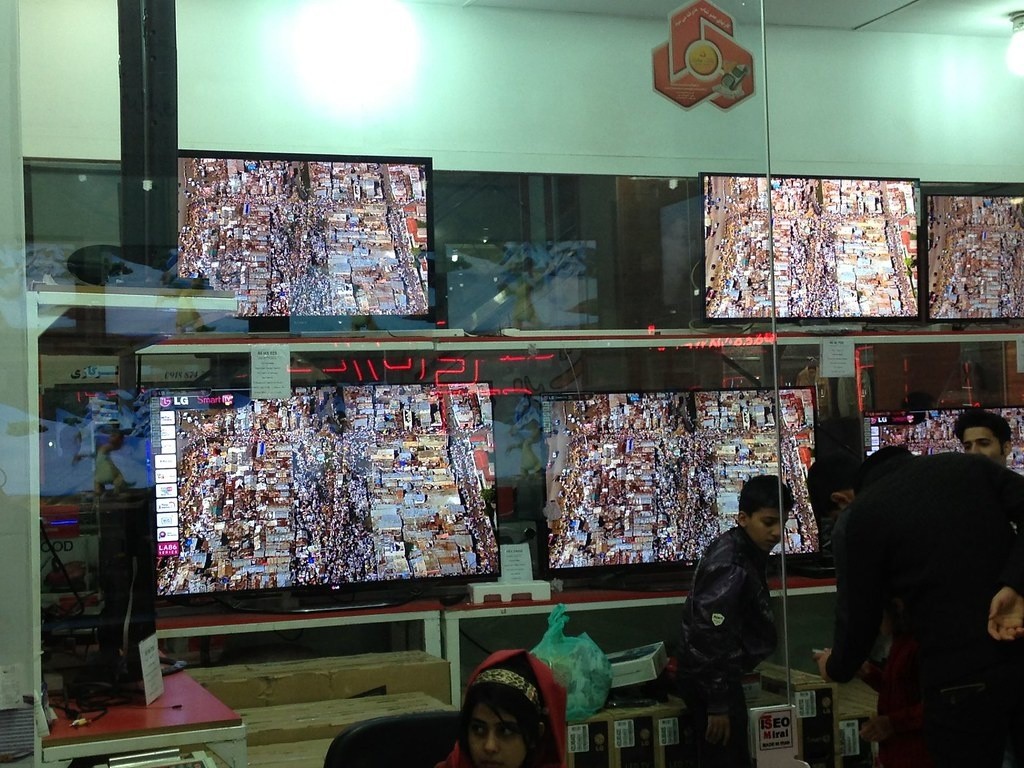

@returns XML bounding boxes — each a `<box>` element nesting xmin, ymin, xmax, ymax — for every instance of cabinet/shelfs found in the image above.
<box><xmin>136</xmin><ymin>333</ymin><xmax>1024</xmax><ymax>705</ymax></box>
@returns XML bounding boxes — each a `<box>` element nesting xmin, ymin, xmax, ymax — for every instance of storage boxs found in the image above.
<box><xmin>183</xmin><ymin>651</ymin><xmax>453</xmax><ymax>711</ymax></box>
<box><xmin>566</xmin><ymin>642</ymin><xmax>874</xmax><ymax>768</ymax></box>
<box><xmin>234</xmin><ymin>693</ymin><xmax>452</xmax><ymax>745</ymax></box>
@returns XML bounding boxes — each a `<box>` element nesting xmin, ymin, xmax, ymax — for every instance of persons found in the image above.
<box><xmin>42</xmin><ymin>424</ymin><xmax>94</xmax><ymax>499</ymax></box>
<box><xmin>807</xmin><ymin>445</ymin><xmax>1024</xmax><ymax>768</ymax></box>
<box><xmin>97</xmin><ymin>419</ymin><xmax>132</xmax><ymax>487</ymax></box>
<box><xmin>681</xmin><ymin>474</ymin><xmax>795</xmax><ymax>768</ymax></box>
<box><xmin>430</xmin><ymin>649</ymin><xmax>567</xmax><ymax>768</ymax></box>
<box><xmin>952</xmin><ymin>409</ymin><xmax>1012</xmax><ymax>467</ymax></box>
<box><xmin>852</xmin><ymin>598</ymin><xmax>928</xmax><ymax>768</ymax></box>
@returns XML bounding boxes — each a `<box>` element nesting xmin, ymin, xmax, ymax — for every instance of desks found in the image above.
<box><xmin>41</xmin><ymin>672</ymin><xmax>247</xmax><ymax>768</ymax></box>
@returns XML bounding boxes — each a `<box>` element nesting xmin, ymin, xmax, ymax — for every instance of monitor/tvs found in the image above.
<box><xmin>45</xmin><ymin>151</ymin><xmax>1024</xmax><ymax>613</ymax></box>
